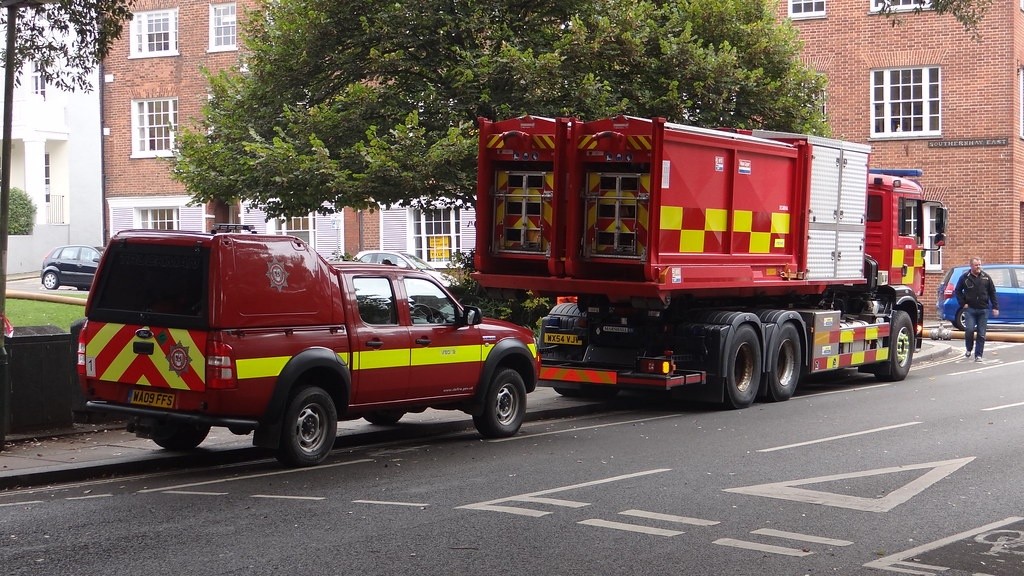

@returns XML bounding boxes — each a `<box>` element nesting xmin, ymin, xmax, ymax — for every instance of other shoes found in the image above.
<box><xmin>965</xmin><ymin>350</ymin><xmax>971</xmax><ymax>358</ymax></box>
<box><xmin>975</xmin><ymin>356</ymin><xmax>983</xmax><ymax>362</ymax></box>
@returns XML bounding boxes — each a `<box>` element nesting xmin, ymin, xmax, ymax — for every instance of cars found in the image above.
<box><xmin>936</xmin><ymin>264</ymin><xmax>1024</xmax><ymax>330</ymax></box>
<box><xmin>40</xmin><ymin>246</ymin><xmax>103</xmax><ymax>291</ymax></box>
<box><xmin>351</xmin><ymin>249</ymin><xmax>461</xmax><ymax>288</ymax></box>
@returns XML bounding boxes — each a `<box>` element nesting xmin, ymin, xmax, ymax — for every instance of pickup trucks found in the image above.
<box><xmin>74</xmin><ymin>225</ymin><xmax>539</xmax><ymax>472</ymax></box>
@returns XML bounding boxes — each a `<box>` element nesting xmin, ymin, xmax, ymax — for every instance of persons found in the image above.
<box><xmin>383</xmin><ymin>260</ymin><xmax>397</xmax><ymax>265</ymax></box>
<box><xmin>955</xmin><ymin>258</ymin><xmax>999</xmax><ymax>362</ymax></box>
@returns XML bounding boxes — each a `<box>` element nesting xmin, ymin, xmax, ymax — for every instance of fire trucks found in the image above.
<box><xmin>469</xmin><ymin>110</ymin><xmax>949</xmax><ymax>410</ymax></box>
